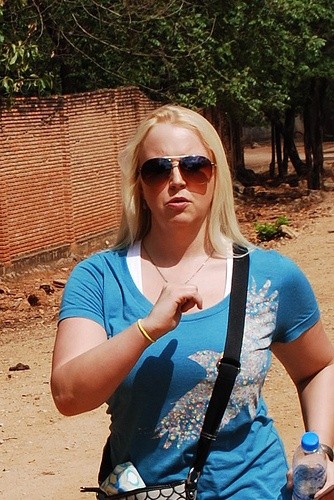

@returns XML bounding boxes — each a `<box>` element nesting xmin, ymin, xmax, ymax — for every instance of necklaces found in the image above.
<box><xmin>140</xmin><ymin>238</ymin><xmax>215</xmax><ymax>285</ymax></box>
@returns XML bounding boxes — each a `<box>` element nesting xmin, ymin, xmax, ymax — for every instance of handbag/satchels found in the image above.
<box><xmin>78</xmin><ymin>438</ymin><xmax>198</xmax><ymax>500</ymax></box>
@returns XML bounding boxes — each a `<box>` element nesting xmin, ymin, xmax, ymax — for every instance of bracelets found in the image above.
<box><xmin>136</xmin><ymin>318</ymin><xmax>156</xmax><ymax>344</ymax></box>
<box><xmin>320</xmin><ymin>444</ymin><xmax>334</xmax><ymax>462</ymax></box>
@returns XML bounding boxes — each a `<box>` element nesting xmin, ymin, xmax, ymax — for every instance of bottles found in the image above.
<box><xmin>292</xmin><ymin>432</ymin><xmax>327</xmax><ymax>500</ymax></box>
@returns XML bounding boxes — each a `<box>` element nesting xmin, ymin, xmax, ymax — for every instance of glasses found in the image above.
<box><xmin>138</xmin><ymin>155</ymin><xmax>216</xmax><ymax>186</ymax></box>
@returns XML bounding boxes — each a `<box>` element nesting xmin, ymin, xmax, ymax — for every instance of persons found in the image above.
<box><xmin>50</xmin><ymin>104</ymin><xmax>334</xmax><ymax>500</ymax></box>
